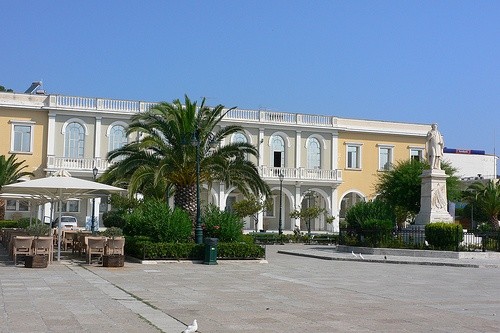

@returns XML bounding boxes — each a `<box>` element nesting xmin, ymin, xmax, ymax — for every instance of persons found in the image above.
<box><xmin>426</xmin><ymin>124</ymin><xmax>443</xmax><ymax>170</ymax></box>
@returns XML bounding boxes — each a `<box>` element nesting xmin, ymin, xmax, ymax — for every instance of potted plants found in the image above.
<box><xmin>25</xmin><ymin>222</ymin><xmax>52</xmax><ymax>268</ymax></box>
<box><xmin>97</xmin><ymin>226</ymin><xmax>124</xmax><ymax>267</ymax></box>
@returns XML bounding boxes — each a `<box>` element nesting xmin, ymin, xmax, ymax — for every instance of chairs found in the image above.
<box><xmin>0</xmin><ymin>227</ymin><xmax>125</xmax><ymax>266</ymax></box>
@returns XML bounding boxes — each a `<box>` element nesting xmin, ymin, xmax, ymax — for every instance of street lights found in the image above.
<box><xmin>195</xmin><ymin>128</ymin><xmax>208</xmax><ymax>245</ymax></box>
<box><xmin>91</xmin><ymin>167</ymin><xmax>99</xmax><ymax>233</ymax></box>
<box><xmin>279</xmin><ymin>174</ymin><xmax>285</xmax><ymax>234</ymax></box>
<box><xmin>305</xmin><ymin>189</ymin><xmax>317</xmax><ymax>235</ymax></box>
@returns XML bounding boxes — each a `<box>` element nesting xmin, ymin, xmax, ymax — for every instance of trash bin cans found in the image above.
<box><xmin>203</xmin><ymin>237</ymin><xmax>219</xmax><ymax>265</ymax></box>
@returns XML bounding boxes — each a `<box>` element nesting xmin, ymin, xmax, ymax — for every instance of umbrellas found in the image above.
<box><xmin>0</xmin><ymin>170</ymin><xmax>129</xmax><ymax>263</ymax></box>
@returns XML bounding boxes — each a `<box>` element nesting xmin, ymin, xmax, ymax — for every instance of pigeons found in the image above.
<box><xmin>359</xmin><ymin>253</ymin><xmax>364</xmax><ymax>260</ymax></box>
<box><xmin>351</xmin><ymin>250</ymin><xmax>357</xmax><ymax>258</ymax></box>
<box><xmin>182</xmin><ymin>319</ymin><xmax>197</xmax><ymax>333</ymax></box>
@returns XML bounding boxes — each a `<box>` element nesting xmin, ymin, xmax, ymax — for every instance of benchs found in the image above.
<box><xmin>306</xmin><ymin>233</ymin><xmax>337</xmax><ymax>245</ymax></box>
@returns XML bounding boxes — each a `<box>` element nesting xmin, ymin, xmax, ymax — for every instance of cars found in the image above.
<box><xmin>44</xmin><ymin>215</ymin><xmax>78</xmax><ymax>230</ymax></box>
